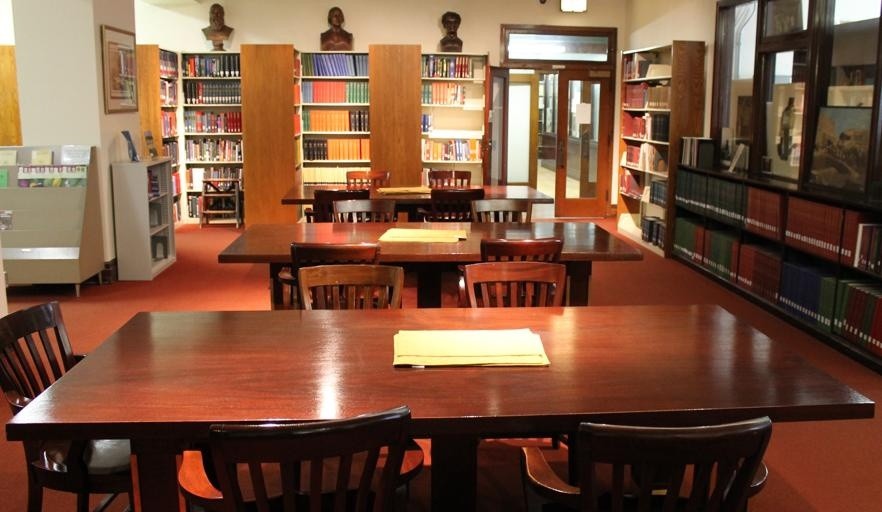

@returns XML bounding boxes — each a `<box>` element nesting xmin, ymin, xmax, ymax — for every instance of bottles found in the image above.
<box><xmin>775</xmin><ymin>96</ymin><xmax>797</xmax><ymax>162</ymax></box>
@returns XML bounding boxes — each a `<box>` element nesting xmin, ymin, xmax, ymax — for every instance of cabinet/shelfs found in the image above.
<box><xmin>240</xmin><ymin>44</ymin><xmax>304</xmax><ymax>230</ymax></box>
<box><xmin>300</xmin><ymin>50</ymin><xmax>369</xmax><ymax>216</ymax></box>
<box><xmin>423</xmin><ymin>53</ymin><xmax>490</xmax><ymax>186</ymax></box>
<box><xmin>183</xmin><ymin>52</ymin><xmax>245</xmax><ymax>223</ymax></box>
<box><xmin>369</xmin><ymin>45</ymin><xmax>423</xmax><ymax>187</ymax></box>
<box><xmin>671</xmin><ymin>166</ymin><xmax>881</xmax><ymax>372</ymax></box>
<box><xmin>112</xmin><ymin>158</ymin><xmax>177</xmax><ymax>281</ymax></box>
<box><xmin>135</xmin><ymin>44</ymin><xmax>182</xmax><ymax>230</ymax></box>
<box><xmin>615</xmin><ymin>40</ymin><xmax>705</xmax><ymax>260</ymax></box>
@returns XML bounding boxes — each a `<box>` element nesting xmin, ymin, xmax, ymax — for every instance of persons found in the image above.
<box><xmin>321</xmin><ymin>8</ymin><xmax>353</xmax><ymax>51</ymax></box>
<box><xmin>202</xmin><ymin>4</ymin><xmax>233</xmax><ymax>41</ymax></box>
<box><xmin>440</xmin><ymin>12</ymin><xmax>463</xmax><ymax>52</ymax></box>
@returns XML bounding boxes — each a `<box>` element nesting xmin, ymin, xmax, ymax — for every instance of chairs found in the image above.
<box><xmin>346</xmin><ymin>170</ymin><xmax>391</xmax><ymax>186</ymax></box>
<box><xmin>1</xmin><ymin>303</ymin><xmax>131</xmax><ymax>512</ymax></box>
<box><xmin>333</xmin><ymin>198</ymin><xmax>397</xmax><ymax>223</ymax></box>
<box><xmin>428</xmin><ymin>171</ymin><xmax>471</xmax><ymax>188</ymax></box>
<box><xmin>178</xmin><ymin>406</ymin><xmax>424</xmax><ymax>512</ymax></box>
<box><xmin>464</xmin><ymin>262</ymin><xmax>566</xmax><ymax>307</ymax></box>
<box><xmin>470</xmin><ymin>198</ymin><xmax>532</xmax><ymax>222</ymax></box>
<box><xmin>277</xmin><ymin>242</ymin><xmax>381</xmax><ymax>310</ymax></box>
<box><xmin>304</xmin><ymin>190</ymin><xmax>370</xmax><ymax>223</ymax></box>
<box><xmin>298</xmin><ymin>265</ymin><xmax>404</xmax><ymax>310</ymax></box>
<box><xmin>417</xmin><ymin>188</ymin><xmax>484</xmax><ymax>222</ymax></box>
<box><xmin>459</xmin><ymin>238</ymin><xmax>564</xmax><ymax>307</ymax></box>
<box><xmin>520</xmin><ymin>414</ymin><xmax>773</xmax><ymax>511</ymax></box>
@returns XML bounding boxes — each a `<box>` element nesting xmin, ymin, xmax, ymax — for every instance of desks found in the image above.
<box><xmin>5</xmin><ymin>302</ymin><xmax>875</xmax><ymax>511</ymax></box>
<box><xmin>218</xmin><ymin>222</ymin><xmax>643</xmax><ymax>310</ymax></box>
<box><xmin>281</xmin><ymin>185</ymin><xmax>555</xmax><ymax>221</ymax></box>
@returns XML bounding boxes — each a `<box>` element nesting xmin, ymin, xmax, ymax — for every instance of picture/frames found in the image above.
<box><xmin>100</xmin><ymin>24</ymin><xmax>138</xmax><ymax>115</ymax></box>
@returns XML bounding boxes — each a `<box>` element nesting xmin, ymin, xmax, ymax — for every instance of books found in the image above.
<box><xmin>0</xmin><ymin>143</ymin><xmax>92</xmax><ymax>189</ymax></box>
<box><xmin>156</xmin><ymin>52</ymin><xmax>242</xmax><ymax>221</ymax></box>
<box><xmin>674</xmin><ymin>170</ymin><xmax>877</xmax><ymax>355</ymax></box>
<box><xmin>421</xmin><ymin>55</ymin><xmax>484</xmax><ymax>162</ymax></box>
<box><xmin>295</xmin><ymin>52</ymin><xmax>369</xmax><ymax>185</ymax></box>
<box><xmin>621</xmin><ymin>52</ymin><xmax>668</xmax><ymax>251</ymax></box>
